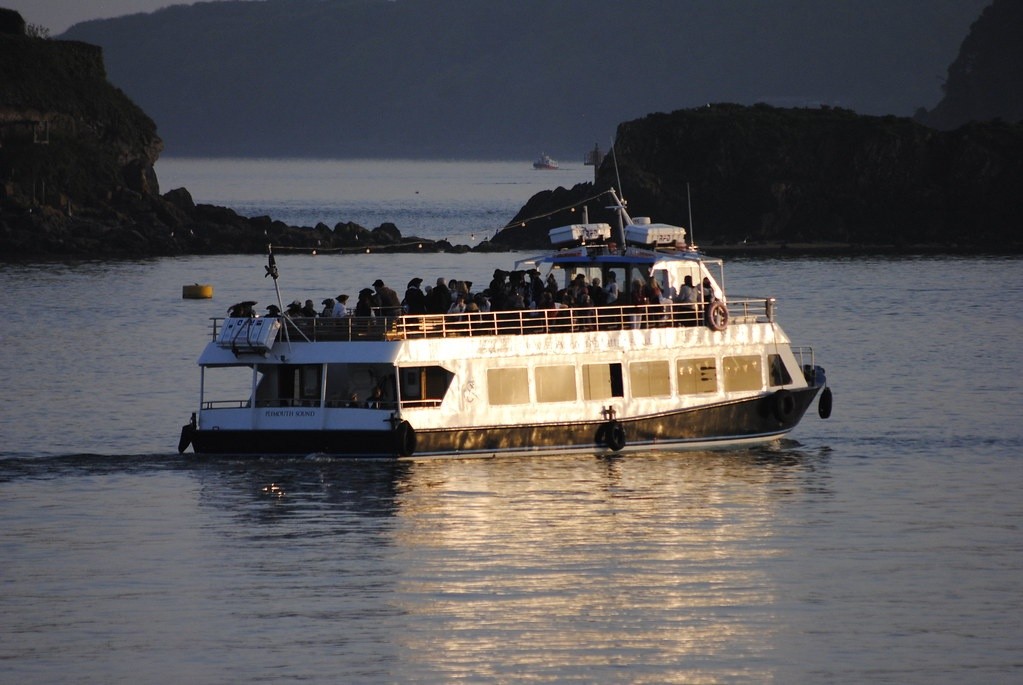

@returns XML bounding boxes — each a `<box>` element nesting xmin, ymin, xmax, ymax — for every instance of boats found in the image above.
<box><xmin>189</xmin><ymin>134</ymin><xmax>827</xmax><ymax>461</ymax></box>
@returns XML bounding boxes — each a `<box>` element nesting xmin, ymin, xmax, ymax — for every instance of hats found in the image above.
<box><xmin>411</xmin><ymin>278</ymin><xmax>424</xmax><ymax>286</ymax></box>
<box><xmin>359</xmin><ymin>288</ymin><xmax>374</xmax><ymax>294</ymax></box>
<box><xmin>287</xmin><ymin>300</ymin><xmax>301</xmax><ymax>306</ymax></box>
<box><xmin>372</xmin><ymin>280</ymin><xmax>384</xmax><ymax>287</ymax></box>
<box><xmin>266</xmin><ymin>305</ymin><xmax>279</xmax><ymax>312</ymax></box>
<box><xmin>527</xmin><ymin>269</ymin><xmax>541</xmax><ymax>274</ymax></box>
<box><xmin>496</xmin><ymin>270</ymin><xmax>509</xmax><ymax>276</ymax></box>
<box><xmin>336</xmin><ymin>295</ymin><xmax>349</xmax><ymax>300</ymax></box>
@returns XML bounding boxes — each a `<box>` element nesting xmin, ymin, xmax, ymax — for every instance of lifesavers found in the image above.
<box><xmin>772</xmin><ymin>389</ymin><xmax>796</xmax><ymax>423</ymax></box>
<box><xmin>818</xmin><ymin>387</ymin><xmax>832</xmax><ymax>419</ymax></box>
<box><xmin>706</xmin><ymin>301</ymin><xmax>731</xmax><ymax>332</ymax></box>
<box><xmin>177</xmin><ymin>423</ymin><xmax>194</xmax><ymax>453</ymax></box>
<box><xmin>397</xmin><ymin>418</ymin><xmax>418</xmax><ymax>456</ymax></box>
<box><xmin>605</xmin><ymin>420</ymin><xmax>627</xmax><ymax>452</ymax></box>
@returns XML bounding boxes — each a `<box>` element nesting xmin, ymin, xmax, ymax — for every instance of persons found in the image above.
<box><xmin>629</xmin><ymin>276</ymin><xmax>665</xmax><ymax>329</ymax></box>
<box><xmin>348</xmin><ymin>393</ymin><xmax>363</xmax><ymax>408</ymax></box>
<box><xmin>365</xmin><ymin>387</ymin><xmax>385</xmax><ymax>409</ymax></box>
<box><xmin>284</xmin><ymin>300</ymin><xmax>305</xmax><ymax>319</ymax></box>
<box><xmin>227</xmin><ymin>301</ymin><xmax>259</xmax><ymax>317</ymax></box>
<box><xmin>697</xmin><ymin>277</ymin><xmax>714</xmax><ymax>310</ymax></box>
<box><xmin>402</xmin><ymin>269</ymin><xmax>619</xmax><ymax>333</ymax></box>
<box><xmin>673</xmin><ymin>275</ymin><xmax>699</xmax><ymax>311</ymax></box>
<box><xmin>322</xmin><ymin>294</ymin><xmax>350</xmax><ymax>326</ymax></box>
<box><xmin>264</xmin><ymin>304</ymin><xmax>282</xmax><ymax>318</ymax></box>
<box><xmin>355</xmin><ymin>279</ymin><xmax>402</xmax><ymax>330</ymax></box>
<box><xmin>302</xmin><ymin>299</ymin><xmax>319</xmax><ymax>317</ymax></box>
